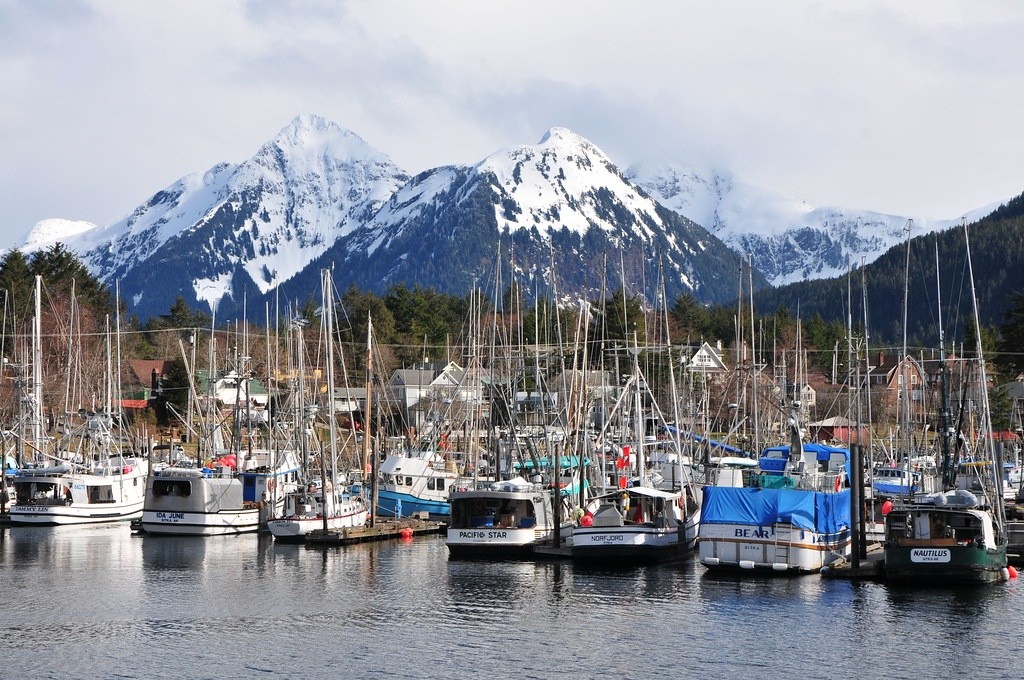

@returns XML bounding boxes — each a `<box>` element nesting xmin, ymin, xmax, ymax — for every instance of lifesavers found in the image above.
<box><xmin>268</xmin><ymin>479</ymin><xmax>275</xmax><ymax>491</ymax></box>
<box><xmin>679</xmin><ymin>497</ymin><xmax>683</xmax><ymax>508</ymax></box>
<box><xmin>547</xmin><ymin>482</ymin><xmax>567</xmax><ymax>488</ymax></box>
<box><xmin>835</xmin><ymin>475</ymin><xmax>841</xmax><ymax>492</ymax></box>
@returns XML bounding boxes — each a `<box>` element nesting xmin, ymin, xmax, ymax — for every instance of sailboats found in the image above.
<box><xmin>0</xmin><ymin>218</ymin><xmax>1024</xmax><ymax>592</ymax></box>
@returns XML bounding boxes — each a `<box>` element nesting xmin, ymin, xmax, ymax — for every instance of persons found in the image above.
<box><xmin>16</xmin><ymin>451</ymin><xmax>25</xmax><ymax>468</ymax></box>
<box><xmin>262</xmin><ymin>490</ymin><xmax>266</xmax><ymax>501</ymax></box>
<box><xmin>839</xmin><ymin>468</ymin><xmax>846</xmax><ymax>490</ymax></box>
<box><xmin>366</xmin><ymin>512</ymin><xmax>371</xmax><ymax>522</ymax></box>
<box><xmin>66</xmin><ymin>486</ymin><xmax>73</xmax><ymax>498</ymax></box>
<box><xmin>969</xmin><ymin>538</ymin><xmax>978</xmax><ymax>548</ymax></box>
<box><xmin>7</xmin><ymin>463</ymin><xmax>12</xmax><ymax>470</ymax></box>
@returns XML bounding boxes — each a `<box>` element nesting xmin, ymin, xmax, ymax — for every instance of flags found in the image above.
<box><xmin>623</xmin><ymin>447</ymin><xmax>629</xmax><ymax>456</ymax></box>
<box><xmin>617</xmin><ymin>458</ymin><xmax>624</xmax><ymax>468</ymax></box>
<box><xmin>624</xmin><ymin>457</ymin><xmax>629</xmax><ymax>466</ymax></box>
